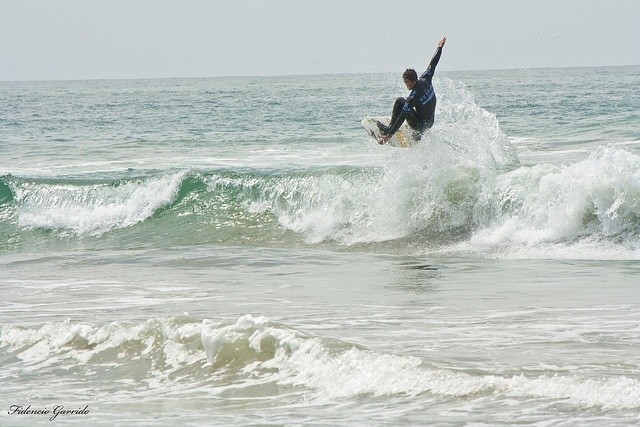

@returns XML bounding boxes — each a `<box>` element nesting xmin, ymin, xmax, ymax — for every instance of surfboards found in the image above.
<box><xmin>361</xmin><ymin>115</ymin><xmax>421</xmax><ymax>148</ymax></box>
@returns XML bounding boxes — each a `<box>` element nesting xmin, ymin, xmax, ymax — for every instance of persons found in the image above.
<box><xmin>379</xmin><ymin>35</ymin><xmax>447</xmax><ymax>145</ymax></box>
